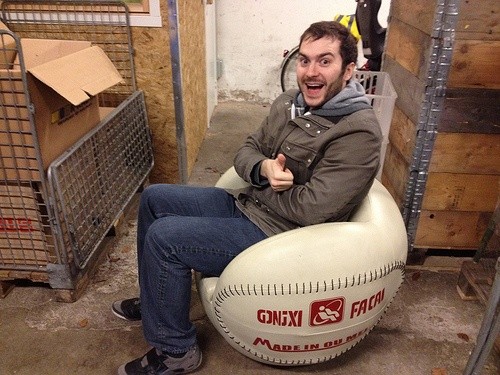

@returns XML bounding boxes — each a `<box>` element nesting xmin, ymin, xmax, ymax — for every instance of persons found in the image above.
<box><xmin>111</xmin><ymin>20</ymin><xmax>385</xmax><ymax>375</ymax></box>
<box><xmin>354</xmin><ymin>0</ymin><xmax>391</xmax><ymax>106</ymax></box>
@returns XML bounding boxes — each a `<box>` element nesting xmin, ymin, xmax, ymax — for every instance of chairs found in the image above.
<box><xmin>197</xmin><ymin>158</ymin><xmax>409</xmax><ymax>369</ymax></box>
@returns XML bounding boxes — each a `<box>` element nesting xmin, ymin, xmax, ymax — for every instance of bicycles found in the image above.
<box><xmin>278</xmin><ymin>46</ymin><xmax>299</xmax><ymax>92</ymax></box>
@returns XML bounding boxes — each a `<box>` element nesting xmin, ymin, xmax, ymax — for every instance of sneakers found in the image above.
<box><xmin>113</xmin><ymin>346</ymin><xmax>204</xmax><ymax>375</ymax></box>
<box><xmin>110</xmin><ymin>296</ymin><xmax>145</xmax><ymax>323</ymax></box>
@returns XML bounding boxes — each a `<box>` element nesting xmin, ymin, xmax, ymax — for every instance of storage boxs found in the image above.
<box><xmin>0</xmin><ymin>20</ymin><xmax>126</xmax><ymax>187</ymax></box>
